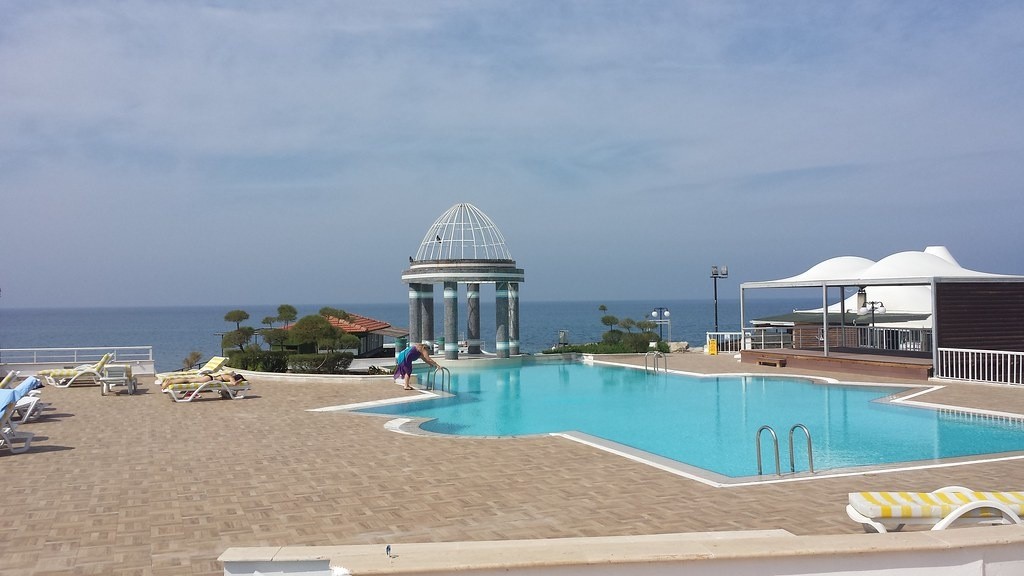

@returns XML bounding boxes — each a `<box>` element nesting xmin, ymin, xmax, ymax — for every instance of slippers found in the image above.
<box><xmin>405</xmin><ymin>387</ymin><xmax>415</xmax><ymax>390</ymax></box>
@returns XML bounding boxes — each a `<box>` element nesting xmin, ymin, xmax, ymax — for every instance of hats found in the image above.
<box><xmin>425</xmin><ymin>341</ymin><xmax>432</xmax><ymax>350</ymax></box>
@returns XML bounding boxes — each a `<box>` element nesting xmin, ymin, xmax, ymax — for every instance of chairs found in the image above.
<box><xmin>36</xmin><ymin>352</ymin><xmax>116</xmax><ymax>388</ymax></box>
<box><xmin>155</xmin><ymin>356</ymin><xmax>251</xmax><ymax>402</ymax></box>
<box><xmin>99</xmin><ymin>363</ymin><xmax>138</xmax><ymax>396</ymax></box>
<box><xmin>0</xmin><ymin>369</ymin><xmax>45</xmax><ymax>455</ymax></box>
<box><xmin>846</xmin><ymin>485</ymin><xmax>1024</xmax><ymax>533</ymax></box>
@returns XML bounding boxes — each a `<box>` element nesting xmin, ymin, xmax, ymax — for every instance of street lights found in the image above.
<box><xmin>860</xmin><ymin>300</ymin><xmax>886</xmax><ymax>347</ymax></box>
<box><xmin>652</xmin><ymin>307</ymin><xmax>670</xmax><ymax>342</ymax></box>
<box><xmin>709</xmin><ymin>265</ymin><xmax>728</xmax><ymax>347</ymax></box>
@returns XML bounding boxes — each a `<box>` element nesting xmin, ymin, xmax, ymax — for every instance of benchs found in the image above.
<box><xmin>756</xmin><ymin>357</ymin><xmax>786</xmax><ymax>368</ymax></box>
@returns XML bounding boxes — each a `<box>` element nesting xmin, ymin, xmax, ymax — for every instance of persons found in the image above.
<box><xmin>393</xmin><ymin>342</ymin><xmax>440</xmax><ymax>390</ymax></box>
<box><xmin>160</xmin><ymin>373</ymin><xmax>248</xmax><ymax>389</ymax></box>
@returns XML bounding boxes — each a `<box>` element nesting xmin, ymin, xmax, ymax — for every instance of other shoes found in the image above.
<box><xmin>186</xmin><ymin>393</ymin><xmax>202</xmax><ymax>398</ymax></box>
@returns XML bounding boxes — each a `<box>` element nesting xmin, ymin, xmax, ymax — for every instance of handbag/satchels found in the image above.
<box><xmin>218</xmin><ymin>390</ymin><xmax>230</xmax><ymax>398</ymax></box>
